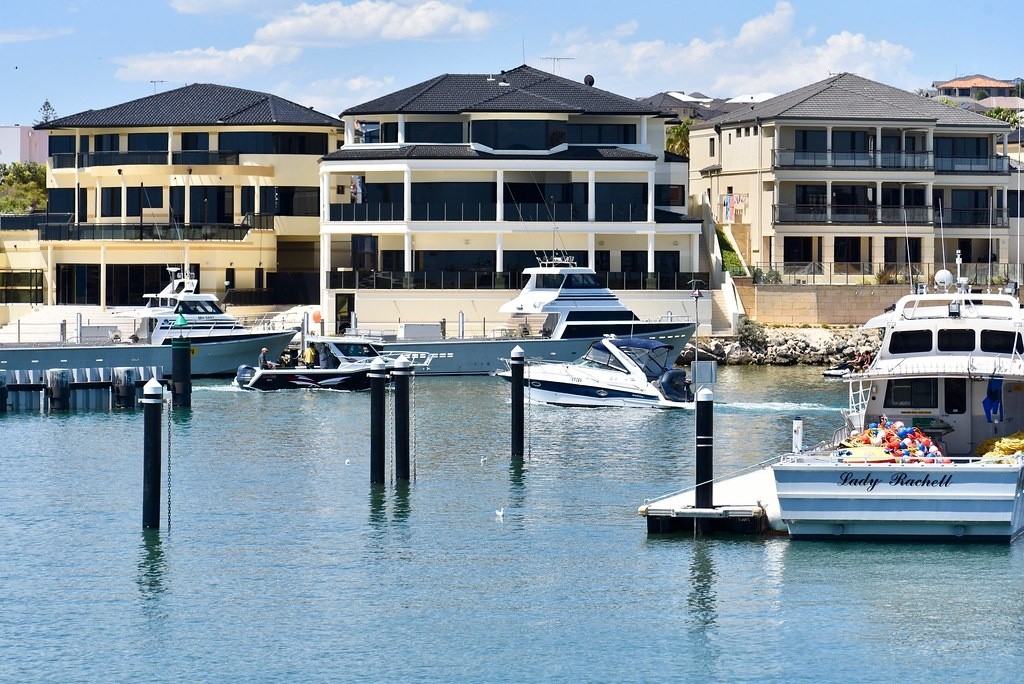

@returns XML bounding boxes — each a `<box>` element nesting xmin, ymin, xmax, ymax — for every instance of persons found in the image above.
<box><xmin>258</xmin><ymin>347</ymin><xmax>273</xmax><ymax>370</ymax></box>
<box><xmin>303</xmin><ymin>343</ymin><xmax>316</xmax><ymax>369</ymax></box>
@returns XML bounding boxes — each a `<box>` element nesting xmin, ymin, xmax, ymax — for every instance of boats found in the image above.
<box><xmin>1</xmin><ymin>180</ymin><xmax>300</xmax><ymax>383</ymax></box>
<box><xmin>485</xmin><ymin>300</ymin><xmax>696</xmax><ymax>412</ymax></box>
<box><xmin>284</xmin><ymin>170</ymin><xmax>702</xmax><ymax>376</ymax></box>
<box><xmin>235</xmin><ymin>311</ymin><xmax>416</xmax><ymax>392</ymax></box>
<box><xmin>771</xmin><ymin>66</ymin><xmax>1024</xmax><ymax>548</ymax></box>
